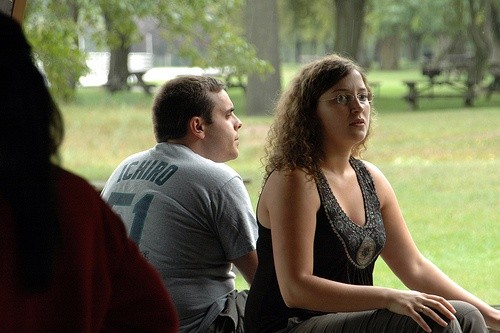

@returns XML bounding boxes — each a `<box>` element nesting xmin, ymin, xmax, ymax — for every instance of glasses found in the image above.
<box><xmin>318</xmin><ymin>92</ymin><xmax>372</xmax><ymax>104</ymax></box>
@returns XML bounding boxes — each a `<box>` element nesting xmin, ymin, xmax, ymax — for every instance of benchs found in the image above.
<box><xmin>127</xmin><ymin>81</ymin><xmax>156</xmax><ymax>95</ymax></box>
<box><xmin>402</xmin><ymin>91</ymin><xmax>478</xmax><ymax>110</ymax></box>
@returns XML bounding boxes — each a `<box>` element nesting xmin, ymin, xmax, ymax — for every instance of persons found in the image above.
<box><xmin>0</xmin><ymin>9</ymin><xmax>180</xmax><ymax>333</ymax></box>
<box><xmin>101</xmin><ymin>75</ymin><xmax>260</xmax><ymax>333</ymax></box>
<box><xmin>243</xmin><ymin>55</ymin><xmax>500</xmax><ymax>333</ymax></box>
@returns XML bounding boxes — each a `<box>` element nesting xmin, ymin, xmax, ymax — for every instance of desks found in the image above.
<box><xmin>402</xmin><ymin>79</ymin><xmax>476</xmax><ymax>110</ymax></box>
<box><xmin>127</xmin><ymin>71</ymin><xmax>147</xmax><ymax>83</ymax></box>
